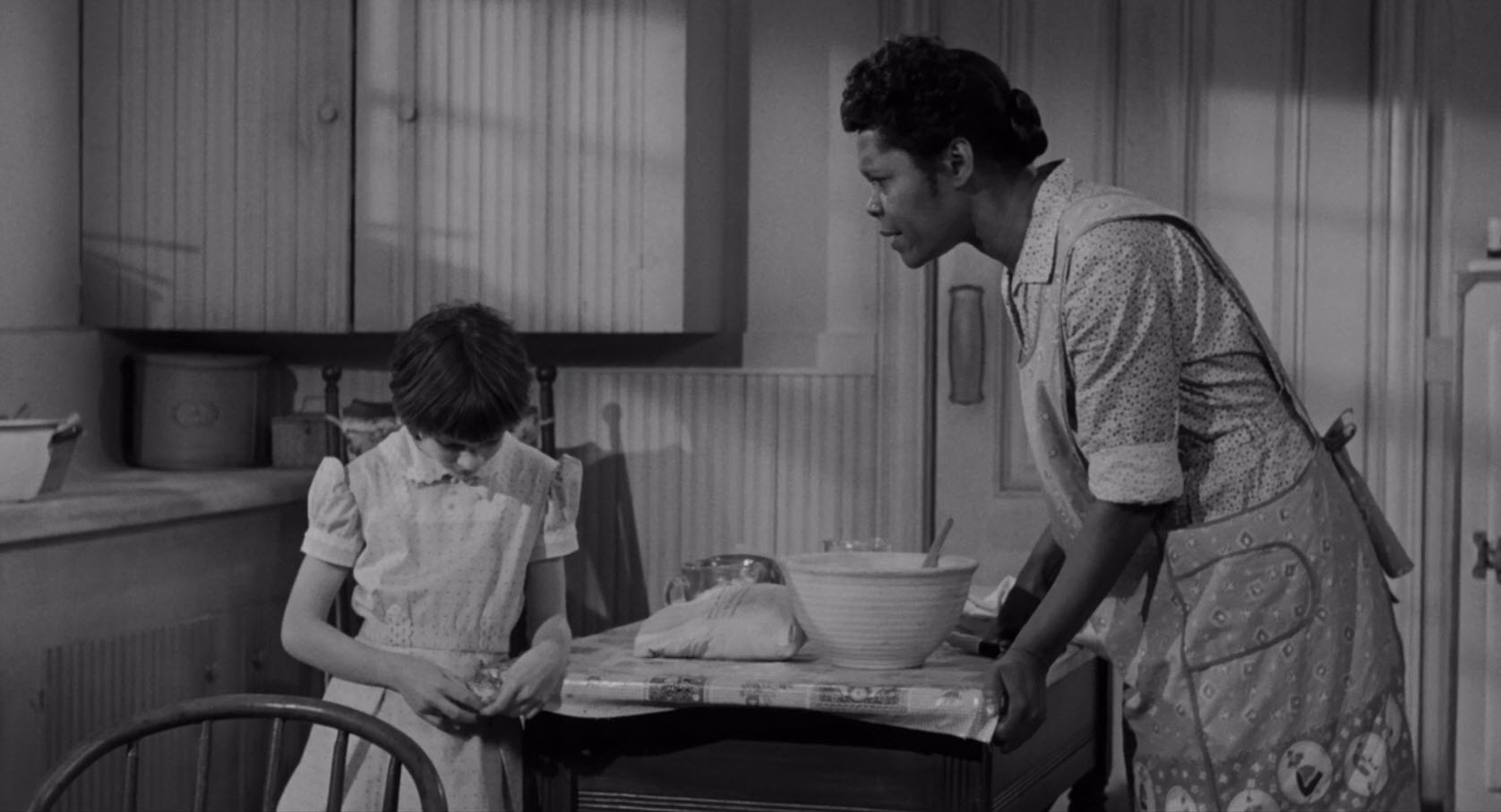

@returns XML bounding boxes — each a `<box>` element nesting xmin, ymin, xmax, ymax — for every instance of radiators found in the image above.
<box><xmin>537</xmin><ymin>359</ymin><xmax>901</xmax><ymax>624</ymax></box>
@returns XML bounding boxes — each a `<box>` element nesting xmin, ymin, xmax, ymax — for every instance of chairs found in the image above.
<box><xmin>30</xmin><ymin>692</ymin><xmax>455</xmax><ymax>812</ymax></box>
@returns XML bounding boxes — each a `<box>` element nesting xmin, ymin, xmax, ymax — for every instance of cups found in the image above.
<box><xmin>680</xmin><ymin>553</ymin><xmax>777</xmax><ymax>602</ymax></box>
<box><xmin>824</xmin><ymin>539</ymin><xmax>891</xmax><ymax>552</ymax></box>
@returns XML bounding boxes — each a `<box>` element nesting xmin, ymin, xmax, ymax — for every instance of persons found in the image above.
<box><xmin>840</xmin><ymin>34</ymin><xmax>1419</xmax><ymax>812</ymax></box>
<box><xmin>276</xmin><ymin>302</ymin><xmax>579</xmax><ymax>812</ymax></box>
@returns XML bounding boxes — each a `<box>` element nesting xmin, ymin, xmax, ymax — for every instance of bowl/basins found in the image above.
<box><xmin>776</xmin><ymin>551</ymin><xmax>980</xmax><ymax>671</ymax></box>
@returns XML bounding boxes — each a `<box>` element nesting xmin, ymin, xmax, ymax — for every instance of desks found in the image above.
<box><xmin>1</xmin><ymin>463</ymin><xmax>329</xmax><ymax>812</ymax></box>
<box><xmin>501</xmin><ymin>580</ymin><xmax>1115</xmax><ymax>812</ymax></box>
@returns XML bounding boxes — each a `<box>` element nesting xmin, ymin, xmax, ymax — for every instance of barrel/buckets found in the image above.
<box><xmin>130</xmin><ymin>348</ymin><xmax>271</xmax><ymax>470</ymax></box>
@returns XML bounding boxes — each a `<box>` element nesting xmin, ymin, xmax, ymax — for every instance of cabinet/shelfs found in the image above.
<box><xmin>76</xmin><ymin>0</ymin><xmax>735</xmax><ymax>348</ymax></box>
